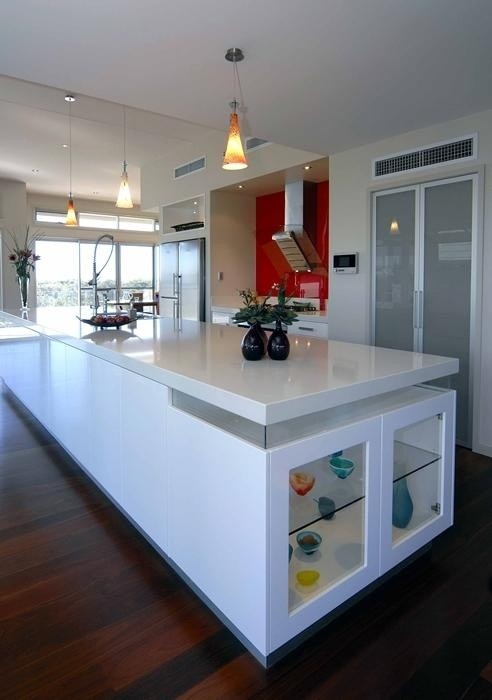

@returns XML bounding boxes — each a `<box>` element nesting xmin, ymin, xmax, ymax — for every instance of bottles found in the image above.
<box><xmin>392</xmin><ymin>478</ymin><xmax>413</xmax><ymax>529</ymax></box>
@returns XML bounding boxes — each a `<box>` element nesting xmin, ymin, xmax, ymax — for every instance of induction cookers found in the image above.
<box><xmin>262</xmin><ymin>304</ymin><xmax>316</xmax><ymax>314</ymax></box>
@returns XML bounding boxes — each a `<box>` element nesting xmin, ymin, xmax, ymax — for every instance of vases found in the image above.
<box><xmin>267</xmin><ymin>318</ymin><xmax>291</xmax><ymax>361</ymax></box>
<box><xmin>392</xmin><ymin>460</ymin><xmax>420</xmax><ymax>530</ymax></box>
<box><xmin>241</xmin><ymin>318</ymin><xmax>269</xmax><ymax>361</ymax></box>
<box><xmin>14</xmin><ymin>274</ymin><xmax>32</xmax><ymax>315</ymax></box>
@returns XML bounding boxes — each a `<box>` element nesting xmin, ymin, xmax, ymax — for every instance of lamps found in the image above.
<box><xmin>112</xmin><ymin>106</ymin><xmax>138</xmax><ymax>212</ymax></box>
<box><xmin>214</xmin><ymin>45</ymin><xmax>254</xmax><ymax>173</ymax></box>
<box><xmin>59</xmin><ymin>94</ymin><xmax>86</xmax><ymax>226</ymax></box>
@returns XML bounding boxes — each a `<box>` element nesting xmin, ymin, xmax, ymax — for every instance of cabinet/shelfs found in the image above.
<box><xmin>211</xmin><ymin>306</ymin><xmax>330</xmax><ymax>342</ymax></box>
<box><xmin>371</xmin><ymin>166</ymin><xmax>477</xmax><ymax>452</ymax></box>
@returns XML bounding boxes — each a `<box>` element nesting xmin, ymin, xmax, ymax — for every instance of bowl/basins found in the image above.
<box><xmin>327</xmin><ymin>457</ymin><xmax>355</xmax><ymax>480</ymax></box>
<box><xmin>296</xmin><ymin>570</ymin><xmax>320</xmax><ymax>584</ymax></box>
<box><xmin>296</xmin><ymin>532</ymin><xmax>323</xmax><ymax>554</ymax></box>
<box><xmin>289</xmin><ymin>473</ymin><xmax>315</xmax><ymax>495</ymax></box>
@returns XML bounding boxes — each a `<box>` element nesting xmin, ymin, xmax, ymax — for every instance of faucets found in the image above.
<box><xmin>102</xmin><ymin>293</ymin><xmax>109</xmax><ymax>312</ymax></box>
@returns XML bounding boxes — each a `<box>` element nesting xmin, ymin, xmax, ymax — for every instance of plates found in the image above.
<box><xmin>81</xmin><ymin>330</ymin><xmax>137</xmax><ymax>339</ymax></box>
<box><xmin>80</xmin><ymin>318</ymin><xmax>138</xmax><ymax>327</ymax></box>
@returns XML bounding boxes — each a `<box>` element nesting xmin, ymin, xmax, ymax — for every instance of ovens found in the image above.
<box><xmin>237</xmin><ymin>321</ymin><xmax>287</xmax><ymax>334</ymax></box>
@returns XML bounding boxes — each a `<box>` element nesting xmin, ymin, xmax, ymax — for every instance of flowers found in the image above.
<box><xmin>268</xmin><ymin>282</ymin><xmax>301</xmax><ymax>325</ymax></box>
<box><xmin>230</xmin><ymin>286</ymin><xmax>276</xmax><ymax>326</ymax></box>
<box><xmin>1</xmin><ymin>221</ymin><xmax>48</xmax><ymax>306</ymax></box>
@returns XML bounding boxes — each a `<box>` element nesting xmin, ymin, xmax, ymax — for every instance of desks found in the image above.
<box><xmin>0</xmin><ymin>303</ymin><xmax>463</xmax><ymax>675</ymax></box>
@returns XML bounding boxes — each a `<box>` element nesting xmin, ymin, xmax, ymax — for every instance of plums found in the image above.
<box><xmin>94</xmin><ymin>315</ymin><xmax>129</xmax><ymax>323</ymax></box>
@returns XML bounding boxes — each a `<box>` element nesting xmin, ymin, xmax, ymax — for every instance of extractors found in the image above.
<box><xmin>271</xmin><ymin>180</ymin><xmax>323</xmax><ymax>273</ymax></box>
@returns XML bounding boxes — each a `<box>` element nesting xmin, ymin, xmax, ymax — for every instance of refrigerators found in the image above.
<box><xmin>158</xmin><ymin>238</ymin><xmax>206</xmax><ymax>323</ymax></box>
